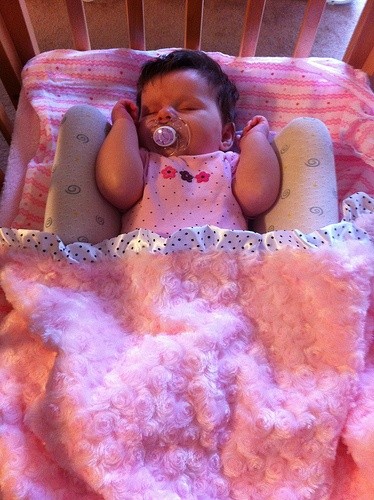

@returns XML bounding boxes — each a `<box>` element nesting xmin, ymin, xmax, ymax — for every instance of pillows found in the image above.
<box><xmin>46</xmin><ymin>106</ymin><xmax>337</xmax><ymax>233</ymax></box>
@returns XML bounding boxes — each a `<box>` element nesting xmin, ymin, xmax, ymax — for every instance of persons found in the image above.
<box><xmin>94</xmin><ymin>48</ymin><xmax>283</xmax><ymax>239</ymax></box>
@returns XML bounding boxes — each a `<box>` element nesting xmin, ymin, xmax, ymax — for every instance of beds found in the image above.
<box><xmin>0</xmin><ymin>49</ymin><xmax>374</xmax><ymax>499</ymax></box>
